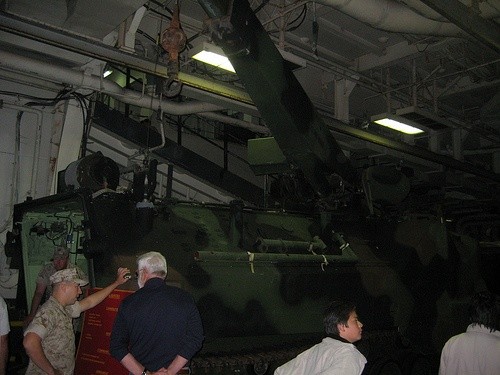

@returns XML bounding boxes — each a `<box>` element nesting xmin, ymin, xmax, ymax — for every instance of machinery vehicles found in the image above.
<box><xmin>0</xmin><ymin>0</ymin><xmax>500</xmax><ymax>375</ymax></box>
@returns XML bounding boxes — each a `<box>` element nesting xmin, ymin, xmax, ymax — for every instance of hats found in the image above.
<box><xmin>49</xmin><ymin>268</ymin><xmax>88</xmax><ymax>287</ymax></box>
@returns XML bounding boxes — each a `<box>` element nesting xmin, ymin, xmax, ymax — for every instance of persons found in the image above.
<box><xmin>0</xmin><ymin>294</ymin><xmax>11</xmax><ymax>375</ymax></box>
<box><xmin>437</xmin><ymin>280</ymin><xmax>500</xmax><ymax>375</ymax></box>
<box><xmin>27</xmin><ymin>248</ymin><xmax>90</xmax><ymax>329</ymax></box>
<box><xmin>273</xmin><ymin>302</ymin><xmax>367</xmax><ymax>375</ymax></box>
<box><xmin>21</xmin><ymin>268</ymin><xmax>130</xmax><ymax>375</ymax></box>
<box><xmin>109</xmin><ymin>252</ymin><xmax>205</xmax><ymax>375</ymax></box>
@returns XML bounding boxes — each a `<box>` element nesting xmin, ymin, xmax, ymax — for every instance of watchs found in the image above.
<box><xmin>142</xmin><ymin>368</ymin><xmax>148</xmax><ymax>375</ymax></box>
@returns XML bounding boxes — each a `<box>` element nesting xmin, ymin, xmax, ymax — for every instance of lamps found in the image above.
<box><xmin>190</xmin><ymin>42</ymin><xmax>236</xmax><ymax>74</ymax></box>
<box><xmin>370</xmin><ymin>113</ymin><xmax>434</xmax><ymax>135</ymax></box>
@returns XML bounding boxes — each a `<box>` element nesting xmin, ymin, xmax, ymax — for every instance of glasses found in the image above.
<box><xmin>134</xmin><ymin>270</ymin><xmax>141</xmax><ymax>278</ymax></box>
